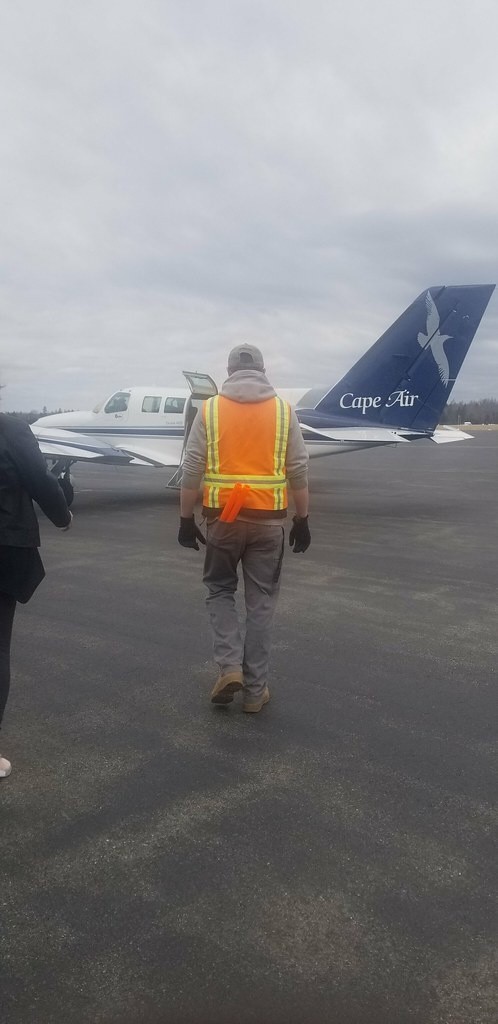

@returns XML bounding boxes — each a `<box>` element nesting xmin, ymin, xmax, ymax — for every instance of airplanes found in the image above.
<box><xmin>28</xmin><ymin>284</ymin><xmax>498</xmax><ymax>511</ymax></box>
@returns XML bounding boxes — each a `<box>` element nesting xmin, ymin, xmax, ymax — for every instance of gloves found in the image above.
<box><xmin>289</xmin><ymin>513</ymin><xmax>311</xmax><ymax>553</ymax></box>
<box><xmin>178</xmin><ymin>513</ymin><xmax>206</xmax><ymax>551</ymax></box>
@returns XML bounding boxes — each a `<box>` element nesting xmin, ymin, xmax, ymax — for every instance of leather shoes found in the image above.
<box><xmin>244</xmin><ymin>686</ymin><xmax>269</xmax><ymax>712</ymax></box>
<box><xmin>211</xmin><ymin>672</ymin><xmax>243</xmax><ymax>703</ymax></box>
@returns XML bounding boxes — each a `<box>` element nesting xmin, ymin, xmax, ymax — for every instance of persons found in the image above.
<box><xmin>178</xmin><ymin>343</ymin><xmax>313</xmax><ymax>713</ymax></box>
<box><xmin>0</xmin><ymin>409</ymin><xmax>75</xmax><ymax>779</ymax></box>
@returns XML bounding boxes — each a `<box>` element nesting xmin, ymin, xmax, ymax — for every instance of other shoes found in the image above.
<box><xmin>0</xmin><ymin>757</ymin><xmax>12</xmax><ymax>777</ymax></box>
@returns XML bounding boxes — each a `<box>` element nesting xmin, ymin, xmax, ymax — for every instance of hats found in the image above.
<box><xmin>228</xmin><ymin>343</ymin><xmax>264</xmax><ymax>371</ymax></box>
